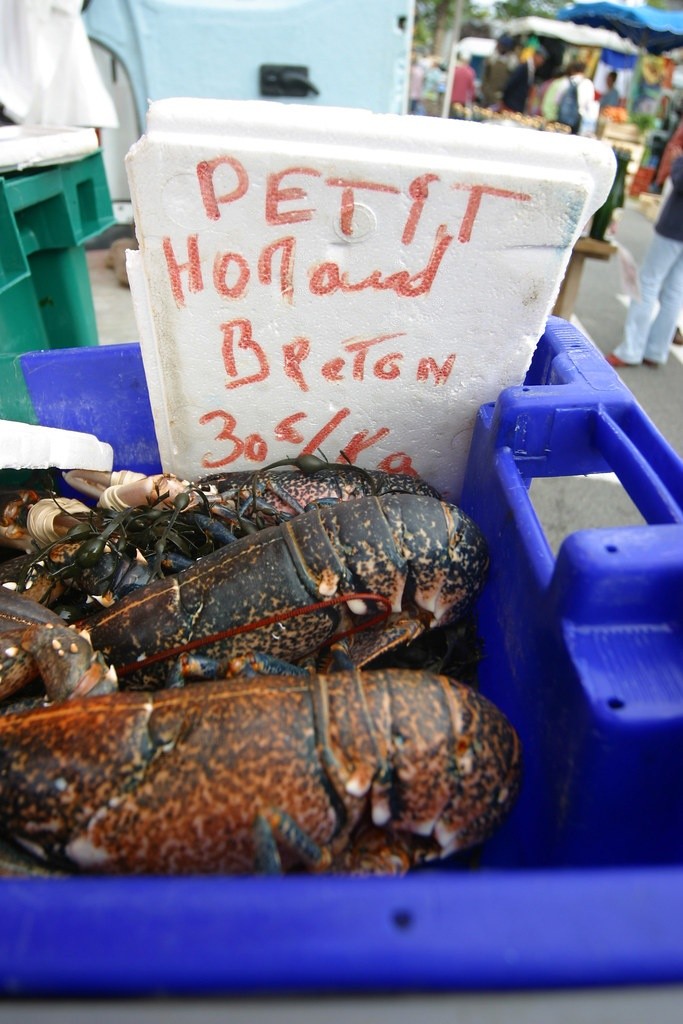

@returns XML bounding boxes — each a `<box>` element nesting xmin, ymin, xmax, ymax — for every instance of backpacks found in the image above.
<box><xmin>557</xmin><ymin>76</ymin><xmax>585</xmax><ymax>127</ymax></box>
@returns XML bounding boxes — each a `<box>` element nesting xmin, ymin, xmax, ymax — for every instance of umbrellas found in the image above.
<box><xmin>558</xmin><ymin>1</ymin><xmax>683</xmax><ymax>56</ymax></box>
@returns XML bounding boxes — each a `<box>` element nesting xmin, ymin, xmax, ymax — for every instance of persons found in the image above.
<box><xmin>411</xmin><ymin>31</ymin><xmax>683</xmax><ymax>369</ymax></box>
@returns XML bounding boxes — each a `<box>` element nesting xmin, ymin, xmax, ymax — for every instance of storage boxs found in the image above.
<box><xmin>0</xmin><ymin>313</ymin><xmax>683</xmax><ymax>994</ymax></box>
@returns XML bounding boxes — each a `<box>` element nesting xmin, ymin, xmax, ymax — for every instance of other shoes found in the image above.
<box><xmin>642</xmin><ymin>358</ymin><xmax>658</xmax><ymax>368</ymax></box>
<box><xmin>605</xmin><ymin>354</ymin><xmax>637</xmax><ymax>367</ymax></box>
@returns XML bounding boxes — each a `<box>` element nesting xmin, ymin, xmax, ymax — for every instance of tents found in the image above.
<box><xmin>454</xmin><ymin>36</ymin><xmax>498</xmax><ymax>86</ymax></box>
<box><xmin>494</xmin><ymin>16</ymin><xmax>639</xmax><ymax>112</ymax></box>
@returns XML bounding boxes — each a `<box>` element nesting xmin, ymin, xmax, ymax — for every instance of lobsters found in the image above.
<box><xmin>1</xmin><ymin>448</ymin><xmax>521</xmax><ymax>880</ymax></box>
